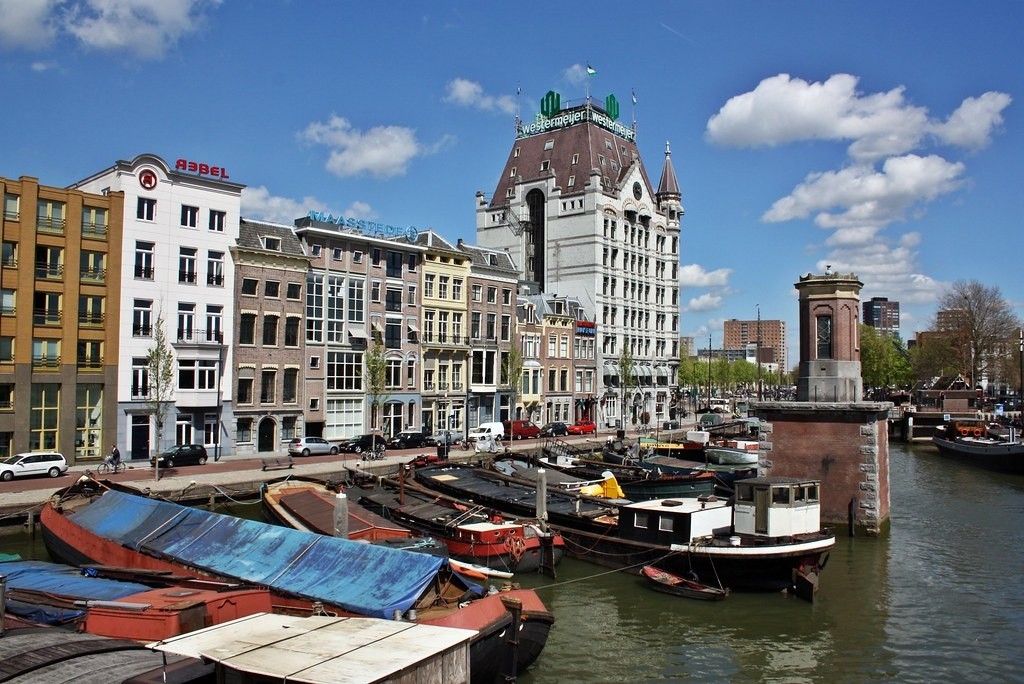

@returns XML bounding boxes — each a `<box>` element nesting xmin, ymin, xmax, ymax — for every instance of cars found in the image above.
<box><xmin>386</xmin><ymin>431</ymin><xmax>427</xmax><ymax>449</ymax></box>
<box><xmin>288</xmin><ymin>437</ymin><xmax>339</xmax><ymax>456</ymax></box>
<box><xmin>338</xmin><ymin>434</ymin><xmax>388</xmax><ymax>454</ymax></box>
<box><xmin>540</xmin><ymin>422</ymin><xmax>569</xmax><ymax>437</ymax></box>
<box><xmin>733</xmin><ymin>391</ymin><xmax>786</xmax><ymax>397</ymax></box>
<box><xmin>566</xmin><ymin>420</ymin><xmax>596</xmax><ymax>435</ymax></box>
<box><xmin>425</xmin><ymin>428</ymin><xmax>464</xmax><ymax>447</ymax></box>
<box><xmin>149</xmin><ymin>444</ymin><xmax>208</xmax><ymax>467</ymax></box>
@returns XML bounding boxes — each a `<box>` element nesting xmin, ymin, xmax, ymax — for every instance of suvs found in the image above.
<box><xmin>711</xmin><ymin>399</ymin><xmax>730</xmax><ymax>412</ymax></box>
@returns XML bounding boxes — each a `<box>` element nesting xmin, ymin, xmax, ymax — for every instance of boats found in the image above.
<box><xmin>932</xmin><ymin>416</ymin><xmax>1024</xmax><ymax>475</ymax></box>
<box><xmin>0</xmin><ymin>476</ymin><xmax>554</xmax><ymax>684</ymax></box>
<box><xmin>328</xmin><ymin>467</ymin><xmax>565</xmax><ymax>574</ymax></box>
<box><xmin>404</xmin><ymin>457</ymin><xmax>836</xmax><ymax>594</ymax></box>
<box><xmin>474</xmin><ymin>416</ymin><xmax>759</xmax><ymax>500</ymax></box>
<box><xmin>641</xmin><ymin>564</ymin><xmax>729</xmax><ymax>602</ymax></box>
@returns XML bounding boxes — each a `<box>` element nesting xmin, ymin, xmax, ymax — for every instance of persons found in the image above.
<box><xmin>108</xmin><ymin>444</ymin><xmax>120</xmax><ymax>473</ymax></box>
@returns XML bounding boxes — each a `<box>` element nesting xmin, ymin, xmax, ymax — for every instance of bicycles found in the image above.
<box><xmin>97</xmin><ymin>455</ymin><xmax>125</xmax><ymax>476</ymax></box>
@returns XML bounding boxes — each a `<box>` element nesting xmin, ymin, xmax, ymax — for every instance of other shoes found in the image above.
<box><xmin>112</xmin><ymin>471</ymin><xmax>116</xmax><ymax>472</ymax></box>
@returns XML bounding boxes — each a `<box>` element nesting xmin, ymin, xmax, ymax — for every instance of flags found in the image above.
<box><xmin>632</xmin><ymin>91</ymin><xmax>636</xmax><ymax>105</ymax></box>
<box><xmin>517</xmin><ymin>87</ymin><xmax>521</xmax><ymax>95</ymax></box>
<box><xmin>587</xmin><ymin>64</ymin><xmax>597</xmax><ymax>76</ymax></box>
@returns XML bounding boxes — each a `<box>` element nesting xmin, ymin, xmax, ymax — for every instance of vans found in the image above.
<box><xmin>468</xmin><ymin>421</ymin><xmax>505</xmax><ymax>442</ymax></box>
<box><xmin>0</xmin><ymin>452</ymin><xmax>68</xmax><ymax>479</ymax></box>
<box><xmin>504</xmin><ymin>420</ymin><xmax>541</xmax><ymax>439</ymax></box>
